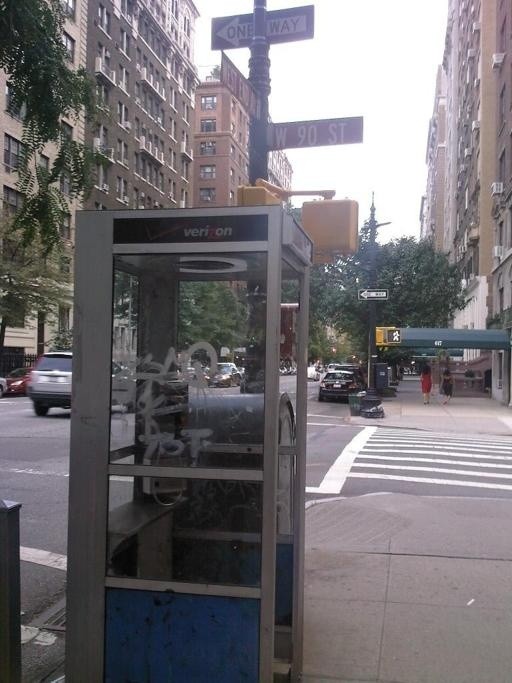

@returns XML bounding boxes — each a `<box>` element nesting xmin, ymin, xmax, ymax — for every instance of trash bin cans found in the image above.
<box><xmin>347</xmin><ymin>392</ymin><xmax>367</xmax><ymax>416</ymax></box>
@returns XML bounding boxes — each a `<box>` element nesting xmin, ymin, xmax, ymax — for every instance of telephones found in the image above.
<box><xmin>134</xmin><ymin>379</ymin><xmax>194</xmax><ymax>506</ymax></box>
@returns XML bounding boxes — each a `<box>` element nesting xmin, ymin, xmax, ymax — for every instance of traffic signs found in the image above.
<box><xmin>358</xmin><ymin>289</ymin><xmax>390</xmax><ymax>301</ymax></box>
<box><xmin>211</xmin><ymin>5</ymin><xmax>314</xmax><ymax>49</ymax></box>
<box><xmin>220</xmin><ymin>50</ymin><xmax>257</xmax><ymax>122</ymax></box>
<box><xmin>267</xmin><ymin>117</ymin><xmax>363</xmax><ymax>151</ymax></box>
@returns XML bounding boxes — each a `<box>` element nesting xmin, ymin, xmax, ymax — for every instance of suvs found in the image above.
<box><xmin>25</xmin><ymin>352</ymin><xmax>136</xmax><ymax>416</ymax></box>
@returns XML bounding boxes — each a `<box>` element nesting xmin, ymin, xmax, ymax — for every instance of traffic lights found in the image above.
<box><xmin>387</xmin><ymin>330</ymin><xmax>402</xmax><ymax>344</ymax></box>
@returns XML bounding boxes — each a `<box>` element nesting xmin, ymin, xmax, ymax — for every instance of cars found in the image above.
<box><xmin>318</xmin><ymin>370</ymin><xmax>362</xmax><ymax>403</ymax></box>
<box><xmin>5</xmin><ymin>368</ymin><xmax>31</xmax><ymax>394</ymax></box>
<box><xmin>205</xmin><ymin>363</ymin><xmax>242</xmax><ymax>388</ymax></box>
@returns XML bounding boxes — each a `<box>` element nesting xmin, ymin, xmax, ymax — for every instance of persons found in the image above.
<box><xmin>405</xmin><ymin>362</ymin><xmax>417</xmax><ymax>376</ymax></box>
<box><xmin>419</xmin><ymin>364</ymin><xmax>434</xmax><ymax>405</ymax></box>
<box><xmin>397</xmin><ymin>364</ymin><xmax>404</xmax><ymax>380</ymax></box>
<box><xmin>439</xmin><ymin>367</ymin><xmax>455</xmax><ymax>404</ymax></box>
